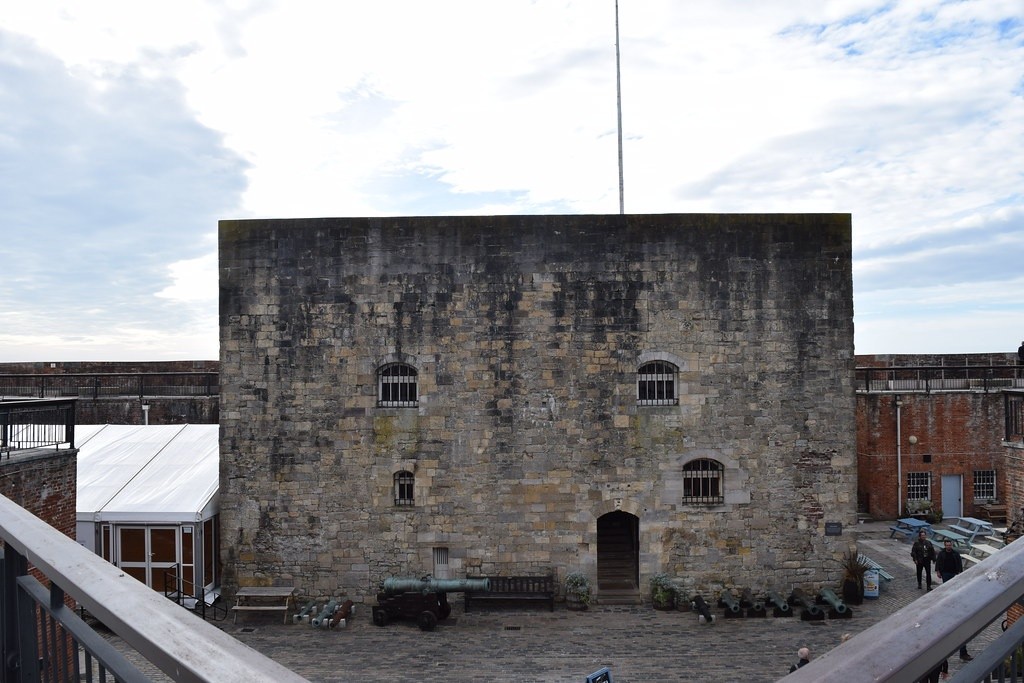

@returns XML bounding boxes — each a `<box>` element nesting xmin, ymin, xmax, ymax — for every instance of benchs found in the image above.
<box><xmin>927</xmin><ymin>538</ymin><xmax>981</xmax><ymax>572</ymax></box>
<box><xmin>879</xmin><ymin>569</ymin><xmax>895</xmax><ymax>583</ymax></box>
<box><xmin>232</xmin><ymin>606</ymin><xmax>290</xmax><ymax>625</ymax></box>
<box><xmin>949</xmin><ymin>520</ymin><xmax>1006</xmax><ymax>548</ymax></box>
<box><xmin>988</xmin><ymin>515</ymin><xmax>1006</xmax><ymax>522</ymax></box>
<box><xmin>463</xmin><ymin>573</ymin><xmax>556</xmax><ymax>613</ymax></box>
<box><xmin>889</xmin><ymin>526</ymin><xmax>918</xmax><ymax>542</ymax></box>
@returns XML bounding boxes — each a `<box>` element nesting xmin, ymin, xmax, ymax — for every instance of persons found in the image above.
<box><xmin>935</xmin><ymin>540</ymin><xmax>964</xmax><ymax>583</ymax></box>
<box><xmin>790</xmin><ymin>648</ymin><xmax>810</xmax><ymax>673</ymax></box>
<box><xmin>909</xmin><ymin>530</ymin><xmax>936</xmax><ymax>591</ymax></box>
<box><xmin>919</xmin><ymin>642</ymin><xmax>974</xmax><ymax>683</ymax></box>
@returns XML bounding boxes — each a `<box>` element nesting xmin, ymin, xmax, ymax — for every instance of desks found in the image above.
<box><xmin>958</xmin><ymin>517</ymin><xmax>995</xmax><ymax>533</ymax></box>
<box><xmin>858</xmin><ymin>554</ymin><xmax>885</xmax><ymax>571</ymax></box>
<box><xmin>969</xmin><ymin>543</ymin><xmax>998</xmax><ymax>557</ymax></box>
<box><xmin>236</xmin><ymin>586</ymin><xmax>296</xmax><ymax>607</ymax></box>
<box><xmin>980</xmin><ymin>505</ymin><xmax>1007</xmax><ymax>514</ymax></box>
<box><xmin>930</xmin><ymin>530</ymin><xmax>971</xmax><ymax>547</ymax></box>
<box><xmin>897</xmin><ymin>517</ymin><xmax>932</xmax><ymax>532</ymax></box>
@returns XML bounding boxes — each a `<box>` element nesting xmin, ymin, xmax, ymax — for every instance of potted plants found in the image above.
<box><xmin>986</xmin><ymin>500</ymin><xmax>994</xmax><ymax>508</ymax></box>
<box><xmin>564</xmin><ymin>571</ymin><xmax>594</xmax><ymax>611</ymax></box>
<box><xmin>841</xmin><ymin>548</ymin><xmax>876</xmax><ymax>604</ymax></box>
<box><xmin>649</xmin><ymin>570</ymin><xmax>693</xmax><ymax>612</ymax></box>
<box><xmin>906</xmin><ymin>497</ymin><xmax>944</xmax><ymax>524</ymax></box>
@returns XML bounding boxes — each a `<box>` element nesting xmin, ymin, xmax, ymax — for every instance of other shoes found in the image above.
<box><xmin>918</xmin><ymin>584</ymin><xmax>922</xmax><ymax>589</ymax></box>
<box><xmin>927</xmin><ymin>587</ymin><xmax>933</xmax><ymax>591</ymax></box>
<box><xmin>961</xmin><ymin>656</ymin><xmax>974</xmax><ymax>662</ymax></box>
